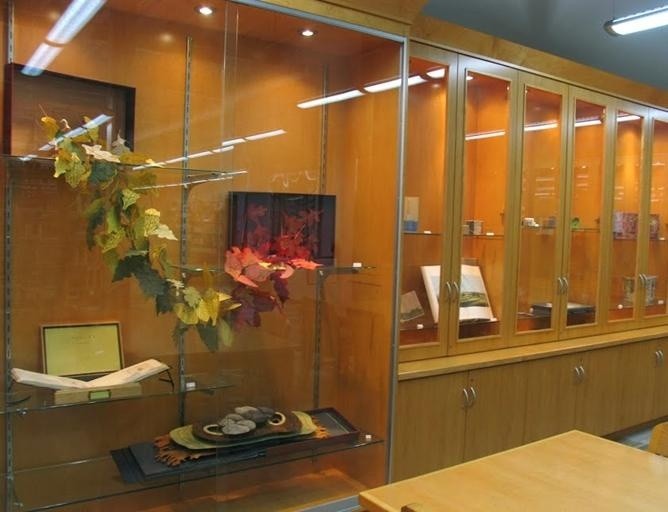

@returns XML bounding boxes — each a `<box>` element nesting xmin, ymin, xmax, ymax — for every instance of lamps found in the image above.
<box><xmin>604</xmin><ymin>5</ymin><xmax>668</xmax><ymax>37</ymax></box>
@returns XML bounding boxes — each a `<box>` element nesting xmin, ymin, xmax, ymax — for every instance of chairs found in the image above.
<box><xmin>649</xmin><ymin>421</ymin><xmax>667</xmax><ymax>458</ymax></box>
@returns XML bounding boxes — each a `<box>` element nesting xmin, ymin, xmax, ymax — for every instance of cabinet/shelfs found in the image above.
<box><xmin>0</xmin><ymin>0</ymin><xmax>431</xmax><ymax>512</ymax></box>
<box><xmin>510</xmin><ymin>39</ymin><xmax>604</xmax><ymax>350</ymax></box>
<box><xmin>528</xmin><ymin>334</ymin><xmax>619</xmax><ymax>444</ymax></box>
<box><xmin>389</xmin><ymin>345</ymin><xmax>526</xmax><ymax>484</ymax></box>
<box><xmin>617</xmin><ymin>325</ymin><xmax>668</xmax><ymax>441</ymax></box>
<box><xmin>396</xmin><ymin>4</ymin><xmax>518</xmax><ymax>369</ymax></box>
<box><xmin>605</xmin><ymin>71</ymin><xmax>668</xmax><ymax>336</ymax></box>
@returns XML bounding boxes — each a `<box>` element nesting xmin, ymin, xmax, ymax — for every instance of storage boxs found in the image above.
<box><xmin>37</xmin><ymin>321</ymin><xmax>142</xmax><ymax>405</ymax></box>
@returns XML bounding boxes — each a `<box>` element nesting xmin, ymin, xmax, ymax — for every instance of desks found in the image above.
<box><xmin>359</xmin><ymin>429</ymin><xmax>668</xmax><ymax>511</ymax></box>
